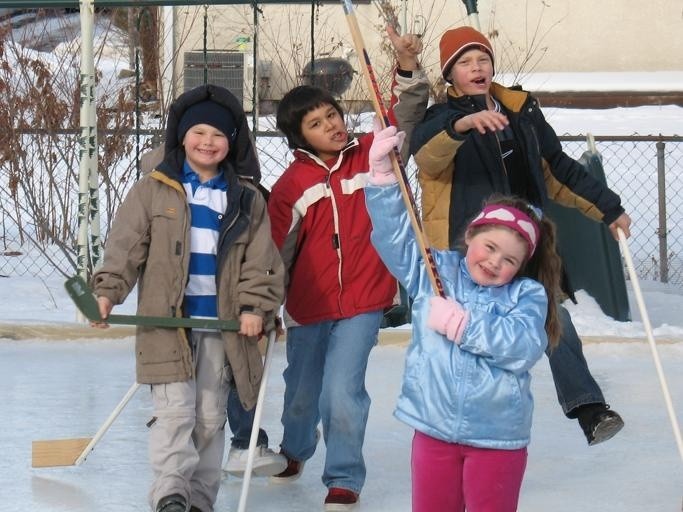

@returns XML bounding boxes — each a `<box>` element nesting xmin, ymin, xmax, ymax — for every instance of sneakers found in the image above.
<box><xmin>577</xmin><ymin>404</ymin><xmax>626</xmax><ymax>448</ymax></box>
<box><xmin>272</xmin><ymin>425</ymin><xmax>322</xmax><ymax>484</ymax></box>
<box><xmin>323</xmin><ymin>484</ymin><xmax>363</xmax><ymax>510</ymax></box>
<box><xmin>222</xmin><ymin>440</ymin><xmax>289</xmax><ymax>479</ymax></box>
<box><xmin>154</xmin><ymin>494</ymin><xmax>205</xmax><ymax>512</ymax></box>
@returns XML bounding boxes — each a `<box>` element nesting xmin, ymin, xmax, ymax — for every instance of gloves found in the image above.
<box><xmin>425</xmin><ymin>294</ymin><xmax>473</xmax><ymax>348</ymax></box>
<box><xmin>366</xmin><ymin>114</ymin><xmax>409</xmax><ymax>188</ymax></box>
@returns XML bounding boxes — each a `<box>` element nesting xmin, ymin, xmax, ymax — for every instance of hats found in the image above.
<box><xmin>438</xmin><ymin>26</ymin><xmax>496</xmax><ymax>86</ymax></box>
<box><xmin>176</xmin><ymin>99</ymin><xmax>239</xmax><ymax>149</ymax></box>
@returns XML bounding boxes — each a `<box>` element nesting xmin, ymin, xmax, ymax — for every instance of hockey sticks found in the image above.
<box><xmin>65</xmin><ymin>275</ymin><xmax>240</xmax><ymax>331</ymax></box>
<box><xmin>32</xmin><ymin>380</ymin><xmax>141</xmax><ymax>467</ymax></box>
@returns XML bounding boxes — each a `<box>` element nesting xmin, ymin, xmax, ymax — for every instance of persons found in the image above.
<box><xmin>363</xmin><ymin>109</ymin><xmax>563</xmax><ymax>512</ymax></box>
<box><xmin>89</xmin><ymin>80</ymin><xmax>283</xmax><ymax>512</ymax></box>
<box><xmin>215</xmin><ymin>316</ymin><xmax>288</xmax><ymax>482</ymax></box>
<box><xmin>269</xmin><ymin>26</ymin><xmax>429</xmax><ymax>512</ymax></box>
<box><xmin>412</xmin><ymin>25</ymin><xmax>631</xmax><ymax>446</ymax></box>
